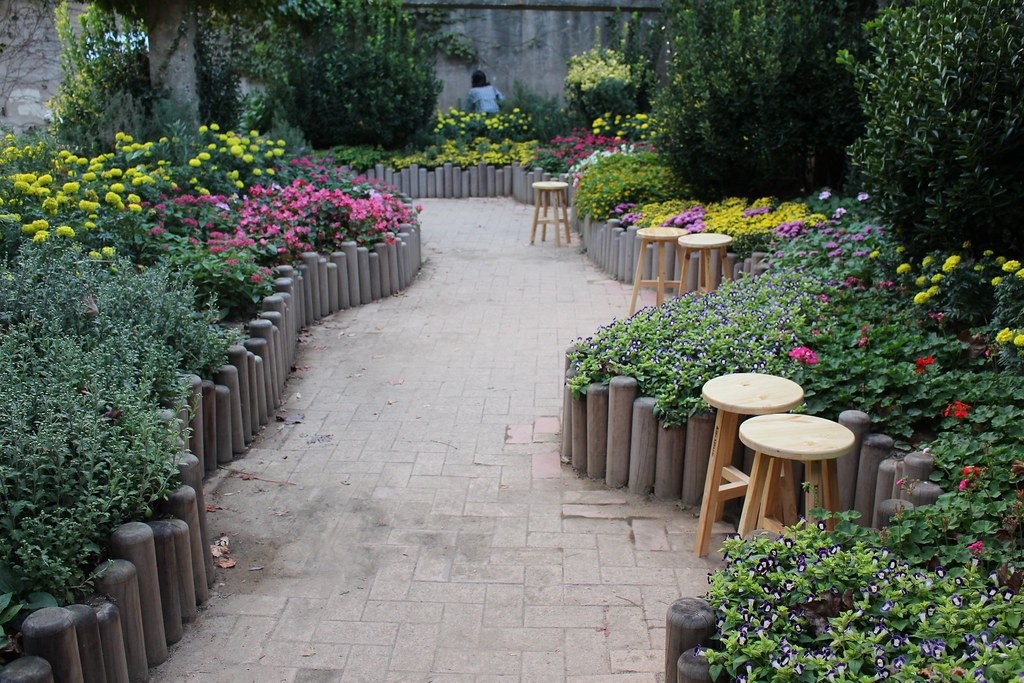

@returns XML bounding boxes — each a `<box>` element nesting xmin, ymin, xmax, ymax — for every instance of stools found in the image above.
<box><xmin>629</xmin><ymin>226</ymin><xmax>687</xmax><ymax>317</ymax></box>
<box><xmin>677</xmin><ymin>233</ymin><xmax>732</xmax><ymax>298</ymax></box>
<box><xmin>529</xmin><ymin>181</ymin><xmax>571</xmax><ymax>247</ymax></box>
<box><xmin>692</xmin><ymin>372</ymin><xmax>805</xmax><ymax>556</ymax></box>
<box><xmin>738</xmin><ymin>412</ymin><xmax>855</xmax><ymax>538</ymax></box>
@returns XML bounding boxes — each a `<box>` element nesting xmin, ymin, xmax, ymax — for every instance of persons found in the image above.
<box><xmin>469</xmin><ymin>70</ymin><xmax>504</xmax><ymax>115</ymax></box>
